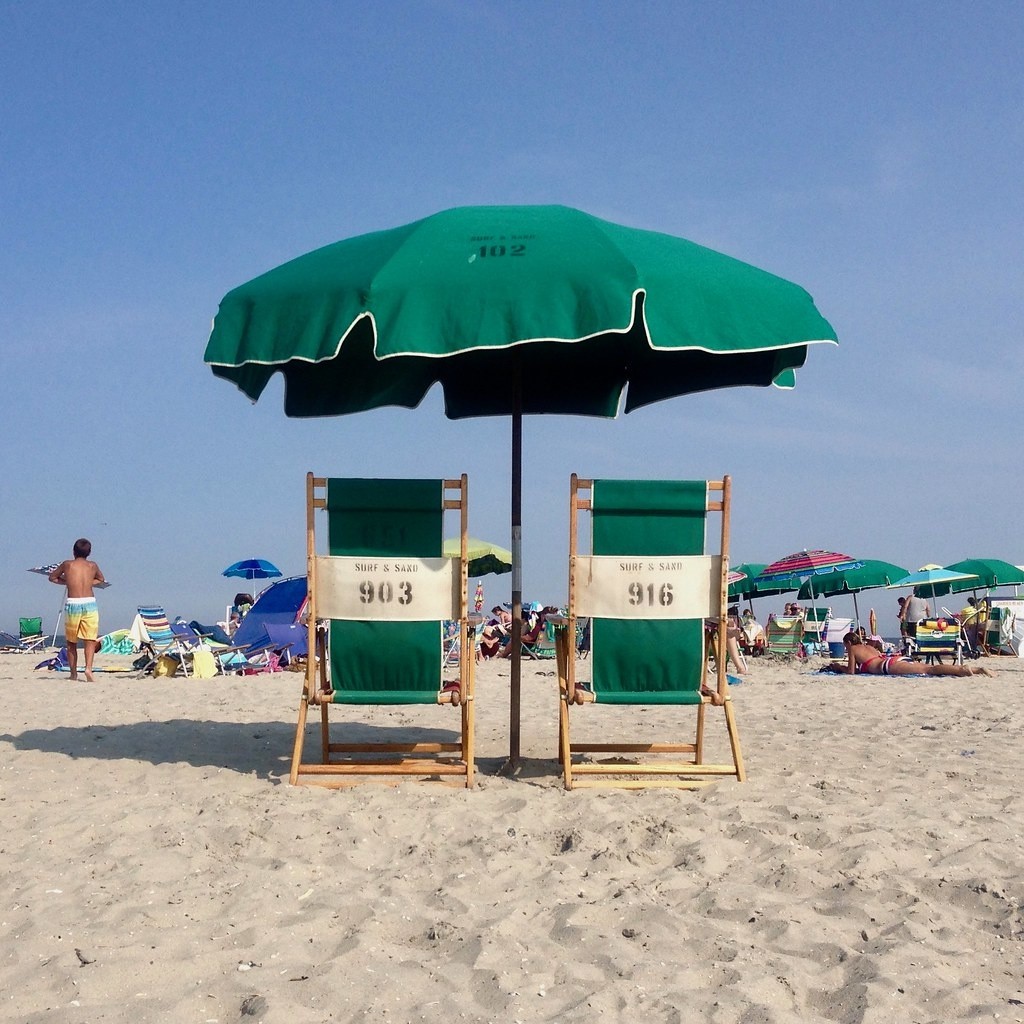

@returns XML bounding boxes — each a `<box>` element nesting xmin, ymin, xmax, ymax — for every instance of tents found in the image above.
<box><xmin>231</xmin><ymin>574</ymin><xmax>307</xmax><ymax>661</ymax></box>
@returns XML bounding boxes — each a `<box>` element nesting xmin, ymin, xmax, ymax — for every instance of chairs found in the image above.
<box><xmin>137</xmin><ymin>604</ymin><xmax>293</xmax><ymax>680</ymax></box>
<box><xmin>803</xmin><ymin>607</ymin><xmax>832</xmax><ymax>656</ymax></box>
<box><xmin>765</xmin><ymin>613</ymin><xmax>804</xmax><ymax>657</ymax></box>
<box><xmin>556</xmin><ymin>474</ymin><xmax>748</xmax><ymax>789</ymax></box>
<box><xmin>983</xmin><ymin>606</ymin><xmax>1018</xmax><ymax>656</ymax></box>
<box><xmin>290</xmin><ymin>473</ymin><xmax>483</xmax><ymax>788</ymax></box>
<box><xmin>518</xmin><ymin>623</ymin><xmax>556</xmax><ymax>660</ymax></box>
<box><xmin>906</xmin><ymin>618</ymin><xmax>962</xmax><ymax>666</ymax></box>
<box><xmin>19</xmin><ymin>617</ymin><xmax>44</xmax><ymax>652</ymax></box>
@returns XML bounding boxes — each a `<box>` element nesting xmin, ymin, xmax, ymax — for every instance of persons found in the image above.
<box><xmin>231</xmin><ymin>613</ymin><xmax>241</xmax><ymax>627</ymax></box>
<box><xmin>829</xmin><ymin>633</ymin><xmax>997</xmax><ymax>677</ymax></box>
<box><xmin>897</xmin><ymin>587</ymin><xmax>931</xmax><ymax>646</ymax></box>
<box><xmin>784</xmin><ymin>603</ymin><xmax>804</xmax><ymax>621</ymax></box>
<box><xmin>456</xmin><ymin>605</ymin><xmax>558</xmax><ymax>660</ymax></box>
<box><xmin>727</xmin><ymin>619</ymin><xmax>752</xmax><ymax>676</ymax></box>
<box><xmin>50</xmin><ymin>538</ymin><xmax>104</xmax><ymax>681</ymax></box>
<box><xmin>727</xmin><ymin>607</ymin><xmax>751</xmax><ymax>654</ymax></box>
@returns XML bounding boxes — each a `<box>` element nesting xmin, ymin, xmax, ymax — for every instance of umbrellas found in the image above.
<box><xmin>871</xmin><ymin>608</ymin><xmax>876</xmax><ymax>635</ymax></box>
<box><xmin>474</xmin><ymin>580</ymin><xmax>484</xmax><ymax>612</ymax></box>
<box><xmin>797</xmin><ymin>559</ymin><xmax>910</xmax><ymax>642</ymax></box>
<box><xmin>221</xmin><ymin>557</ymin><xmax>283</xmax><ymax>600</ymax></box>
<box><xmin>913</xmin><ymin>557</ymin><xmax>1024</xmax><ymax>609</ymax></box>
<box><xmin>753</xmin><ymin>548</ymin><xmax>866</xmax><ymax>642</ymax></box>
<box><xmin>27</xmin><ymin>561</ymin><xmax>111</xmax><ymax>646</ymax></box>
<box><xmin>886</xmin><ymin>564</ymin><xmax>979</xmax><ymax>617</ymax></box>
<box><xmin>205</xmin><ymin>205</ymin><xmax>841</xmax><ymax>780</ymax></box>
<box><xmin>443</xmin><ymin>538</ymin><xmax>512</xmax><ymax>577</ymax></box>
<box><xmin>728</xmin><ymin>563</ymin><xmax>802</xmax><ymax>616</ymax></box>
